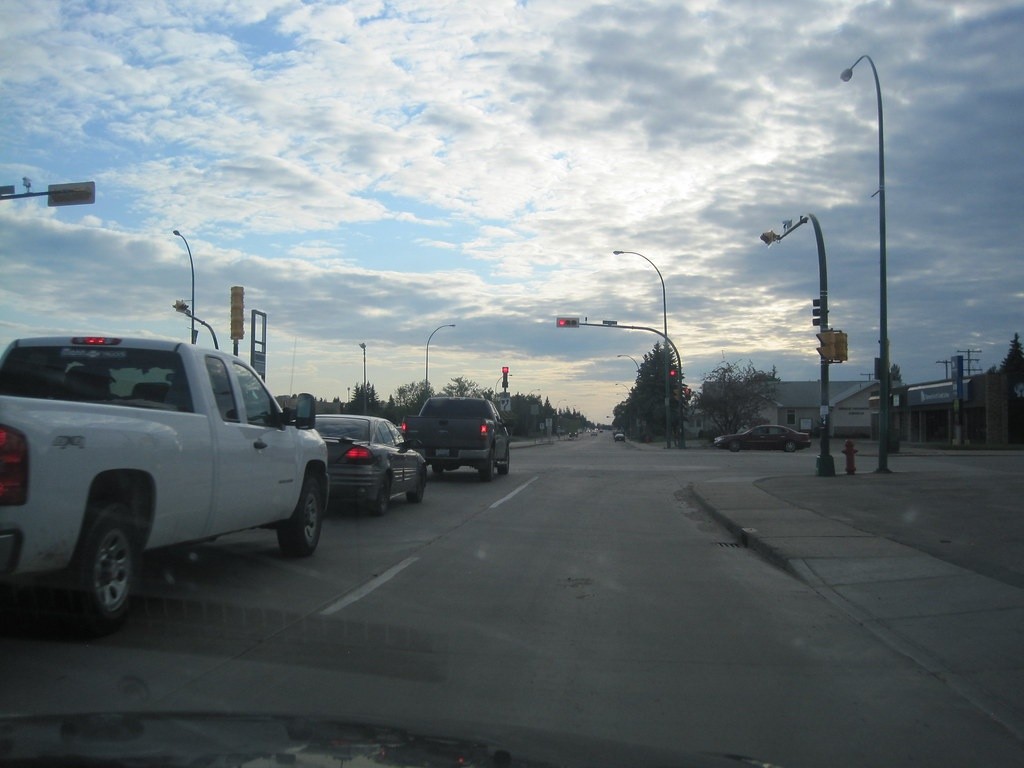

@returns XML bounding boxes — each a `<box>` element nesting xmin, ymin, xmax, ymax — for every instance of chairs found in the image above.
<box><xmin>130</xmin><ymin>380</ymin><xmax>173</xmax><ymax>402</ymax></box>
<box><xmin>63</xmin><ymin>364</ymin><xmax>113</xmax><ymax>401</ymax></box>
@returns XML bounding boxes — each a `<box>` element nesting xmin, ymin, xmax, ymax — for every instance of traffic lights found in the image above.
<box><xmin>761</xmin><ymin>231</ymin><xmax>773</xmax><ymax>246</ymax></box>
<box><xmin>176</xmin><ymin>299</ymin><xmax>189</xmax><ymax>312</ymax></box>
<box><xmin>816</xmin><ymin>331</ymin><xmax>836</xmax><ymax>359</ymax></box>
<box><xmin>556</xmin><ymin>317</ymin><xmax>579</xmax><ymax>329</ymax></box>
<box><xmin>812</xmin><ymin>299</ymin><xmax>821</xmax><ymax>325</ymax></box>
<box><xmin>503</xmin><ymin>368</ymin><xmax>508</xmax><ymax>387</ymax></box>
<box><xmin>669</xmin><ymin>364</ymin><xmax>677</xmax><ymax>388</ymax></box>
<box><xmin>685</xmin><ymin>389</ymin><xmax>690</xmax><ymax>399</ymax></box>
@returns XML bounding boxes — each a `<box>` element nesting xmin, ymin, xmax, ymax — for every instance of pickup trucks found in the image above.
<box><xmin>400</xmin><ymin>399</ymin><xmax>513</xmax><ymax>480</ymax></box>
<box><xmin>1</xmin><ymin>335</ymin><xmax>331</xmax><ymax>630</ymax></box>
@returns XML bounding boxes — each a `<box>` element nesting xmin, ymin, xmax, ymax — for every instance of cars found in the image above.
<box><xmin>569</xmin><ymin>429</ymin><xmax>578</xmax><ymax>437</ymax></box>
<box><xmin>586</xmin><ymin>428</ymin><xmax>604</xmax><ymax>436</ymax></box>
<box><xmin>313</xmin><ymin>414</ymin><xmax>430</xmax><ymax>516</ymax></box>
<box><xmin>614</xmin><ymin>434</ymin><xmax>625</xmax><ymax>442</ymax></box>
<box><xmin>714</xmin><ymin>425</ymin><xmax>813</xmax><ymax>452</ymax></box>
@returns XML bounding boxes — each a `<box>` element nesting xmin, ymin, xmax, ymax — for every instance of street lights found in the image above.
<box><xmin>618</xmin><ymin>354</ymin><xmax>641</xmax><ymax>441</ymax></box>
<box><xmin>425</xmin><ymin>324</ymin><xmax>455</xmax><ymax>397</ymax></box>
<box><xmin>614</xmin><ymin>251</ymin><xmax>672</xmax><ymax>448</ymax></box>
<box><xmin>840</xmin><ymin>54</ymin><xmax>894</xmax><ymax>473</ymax></box>
<box><xmin>615</xmin><ymin>384</ymin><xmax>632</xmax><ymax>440</ymax></box>
<box><xmin>495</xmin><ymin>375</ymin><xmax>513</xmax><ymax>404</ymax></box>
<box><xmin>360</xmin><ymin>342</ymin><xmax>367</xmax><ymax>414</ymax></box>
<box><xmin>173</xmin><ymin>229</ymin><xmax>194</xmax><ymax>342</ymax></box>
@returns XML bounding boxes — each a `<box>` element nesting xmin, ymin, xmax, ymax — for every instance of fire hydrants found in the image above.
<box><xmin>842</xmin><ymin>439</ymin><xmax>859</xmax><ymax>475</ymax></box>
